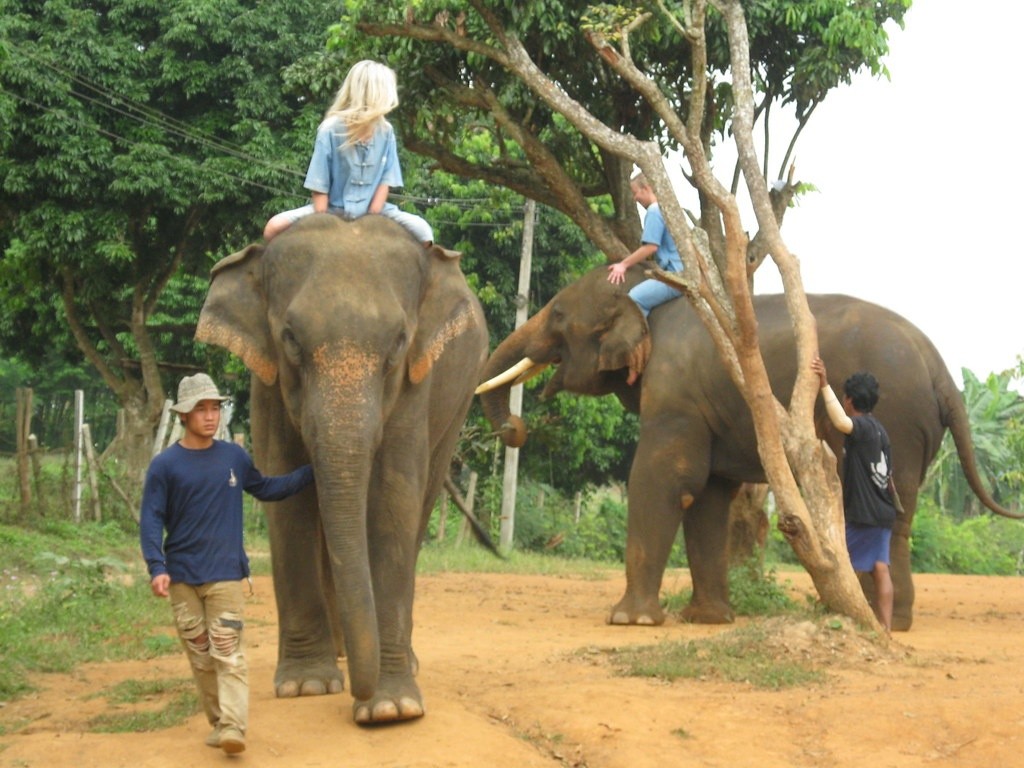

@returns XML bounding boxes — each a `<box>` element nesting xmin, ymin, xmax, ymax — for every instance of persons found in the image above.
<box><xmin>263</xmin><ymin>59</ymin><xmax>435</xmax><ymax>246</ymax></box>
<box><xmin>811</xmin><ymin>358</ymin><xmax>898</xmax><ymax>640</ymax></box>
<box><xmin>605</xmin><ymin>170</ymin><xmax>687</xmax><ymax>386</ymax></box>
<box><xmin>140</xmin><ymin>373</ymin><xmax>314</xmax><ymax>755</ymax></box>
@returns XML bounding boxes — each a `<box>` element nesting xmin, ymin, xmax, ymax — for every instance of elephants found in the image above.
<box><xmin>193</xmin><ymin>211</ymin><xmax>490</xmax><ymax>723</ymax></box>
<box><xmin>474</xmin><ymin>261</ymin><xmax>1023</xmax><ymax>632</ymax></box>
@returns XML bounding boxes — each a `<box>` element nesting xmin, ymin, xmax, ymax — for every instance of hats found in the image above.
<box><xmin>169</xmin><ymin>372</ymin><xmax>228</xmax><ymax>414</ymax></box>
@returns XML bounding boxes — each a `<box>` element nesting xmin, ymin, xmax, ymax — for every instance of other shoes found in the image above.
<box><xmin>219</xmin><ymin>729</ymin><xmax>246</xmax><ymax>753</ymax></box>
<box><xmin>205</xmin><ymin>727</ymin><xmax>222</xmax><ymax>746</ymax></box>
